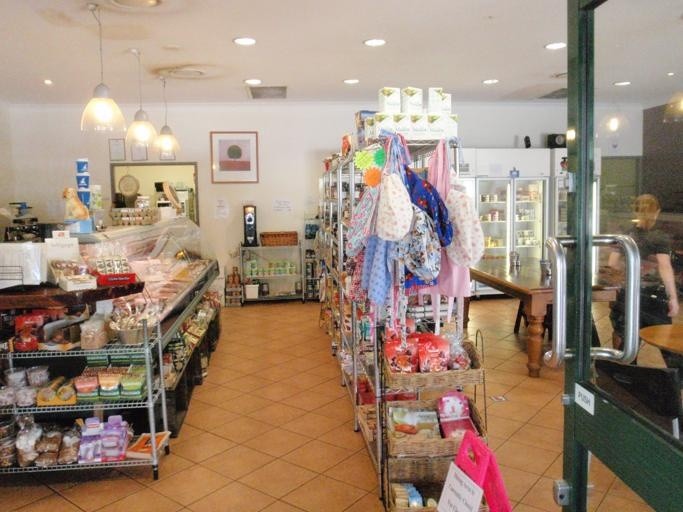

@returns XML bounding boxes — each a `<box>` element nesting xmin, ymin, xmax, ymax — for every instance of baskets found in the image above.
<box><xmin>118</xmin><ymin>316</ymin><xmax>153</xmax><ymax>344</ymax></box>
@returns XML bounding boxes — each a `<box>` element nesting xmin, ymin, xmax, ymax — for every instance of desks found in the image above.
<box><xmin>460</xmin><ymin>256</ymin><xmax>623</xmax><ymax>380</ymax></box>
<box><xmin>637</xmin><ymin>320</ymin><xmax>682</xmax><ymax>374</ymax></box>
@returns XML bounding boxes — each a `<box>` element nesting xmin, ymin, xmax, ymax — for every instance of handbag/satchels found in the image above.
<box><xmin>346</xmin><ymin>165</ymin><xmax>486</xmax><ymax>306</ymax></box>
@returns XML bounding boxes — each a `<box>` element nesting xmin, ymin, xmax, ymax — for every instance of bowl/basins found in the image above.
<box><xmin>95</xmin><ymin>226</ymin><xmax>106</xmax><ymax>231</ymax></box>
<box><xmin>116</xmin><ymin>326</ymin><xmax>153</xmax><ymax>346</ymax></box>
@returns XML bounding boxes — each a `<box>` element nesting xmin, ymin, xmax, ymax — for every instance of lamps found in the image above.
<box><xmin>152</xmin><ymin>77</ymin><xmax>181</xmax><ymax>153</ymax></box>
<box><xmin>125</xmin><ymin>47</ymin><xmax>158</xmax><ymax>143</ymax></box>
<box><xmin>78</xmin><ymin>1</ymin><xmax>127</xmax><ymax>134</ymax></box>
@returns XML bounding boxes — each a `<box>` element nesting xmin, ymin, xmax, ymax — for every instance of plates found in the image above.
<box><xmin>118</xmin><ymin>175</ymin><xmax>138</xmax><ymax>196</ymax></box>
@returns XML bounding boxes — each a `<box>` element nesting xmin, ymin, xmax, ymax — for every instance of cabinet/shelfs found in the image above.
<box><xmin>318</xmin><ymin>139</ymin><xmax>485</xmax><ymax>509</ymax></box>
<box><xmin>0</xmin><ymin>214</ymin><xmax>224</xmax><ymax>485</ymax></box>
<box><xmin>302</xmin><ymin>210</ymin><xmax>320</xmax><ymax>303</ymax></box>
<box><xmin>238</xmin><ymin>239</ymin><xmax>304</xmax><ymax>305</ymax></box>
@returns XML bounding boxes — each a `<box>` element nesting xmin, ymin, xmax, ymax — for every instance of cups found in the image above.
<box><xmin>480</xmin><ymin>210</ymin><xmax>498</xmax><ymax>221</ymax></box>
<box><xmin>481</xmin><ymin>194</ymin><xmax>488</xmax><ymax>202</ymax></box>
<box><xmin>516</xmin><ymin>229</ymin><xmax>537</xmax><ymax>246</ymax></box>
<box><xmin>489</xmin><ymin>195</ymin><xmax>497</xmax><ymax>203</ymax></box>
<box><xmin>560</xmin><ymin>191</ymin><xmax>567</xmax><ymax>201</ymax></box>
<box><xmin>484</xmin><ymin>236</ymin><xmax>503</xmax><ymax>247</ymax></box>
<box><xmin>515</xmin><ymin>208</ymin><xmax>535</xmax><ymax>220</ymax></box>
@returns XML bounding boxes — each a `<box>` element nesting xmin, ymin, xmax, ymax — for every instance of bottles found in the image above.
<box><xmin>134</xmin><ymin>195</ymin><xmax>151</xmax><ymax>208</ymax></box>
<box><xmin>304</xmin><ymin>223</ymin><xmax>317</xmax><ymax>239</ymax></box>
<box><xmin>539</xmin><ymin>260</ymin><xmax>552</xmax><ymax>288</ymax></box>
<box><xmin>509</xmin><ymin>251</ymin><xmax>520</xmax><ymax>266</ymax></box>
<box><xmin>261</xmin><ymin>283</ymin><xmax>268</xmax><ymax>296</ymax></box>
<box><xmin>307</xmin><ymin>284</ymin><xmax>318</xmax><ymax>298</ymax></box>
<box><xmin>560</xmin><ymin>157</ymin><xmax>567</xmax><ymax>169</ymax></box>
<box><xmin>295</xmin><ymin>281</ymin><xmax>301</xmax><ymax>294</ymax></box>
<box><xmin>306</xmin><ymin>262</ymin><xmax>311</xmax><ymax>277</ymax></box>
<box><xmin>510</xmin><ymin>167</ymin><xmax>519</xmax><ymax>178</ymax></box>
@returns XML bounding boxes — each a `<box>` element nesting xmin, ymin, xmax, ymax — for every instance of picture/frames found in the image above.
<box><xmin>130</xmin><ymin>142</ymin><xmax>147</xmax><ymax>162</ymax></box>
<box><xmin>107</xmin><ymin>138</ymin><xmax>126</xmax><ymax>162</ymax></box>
<box><xmin>158</xmin><ymin>152</ymin><xmax>176</xmax><ymax>162</ymax></box>
<box><xmin>209</xmin><ymin>130</ymin><xmax>259</xmax><ymax>185</ymax></box>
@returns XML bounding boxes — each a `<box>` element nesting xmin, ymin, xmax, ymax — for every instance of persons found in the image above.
<box><xmin>609</xmin><ymin>193</ymin><xmax>683</xmax><ymax>368</ymax></box>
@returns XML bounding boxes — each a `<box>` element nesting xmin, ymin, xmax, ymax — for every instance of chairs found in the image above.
<box><xmin>596</xmin><ymin>353</ymin><xmax>682</xmax><ymax>445</ymax></box>
